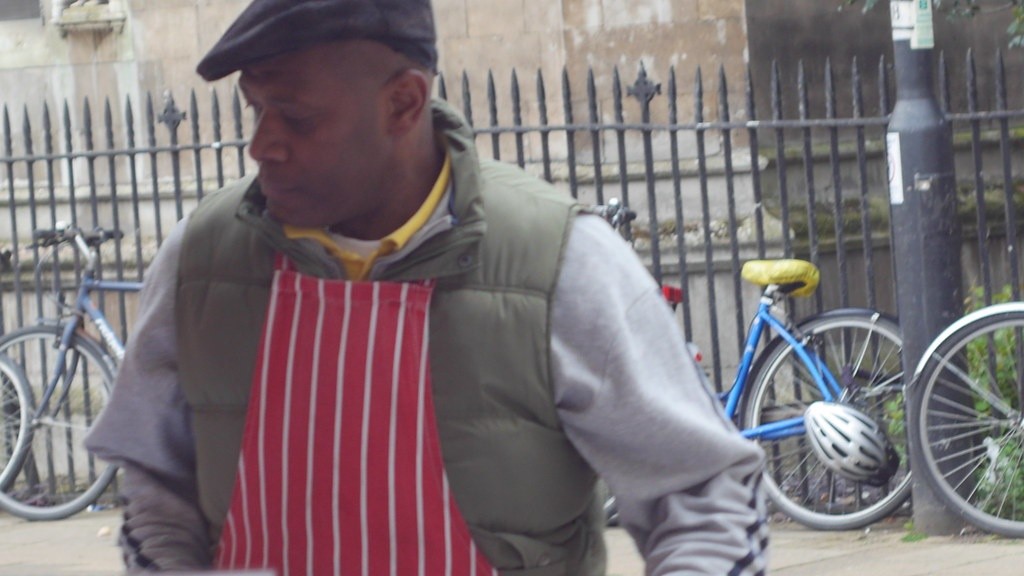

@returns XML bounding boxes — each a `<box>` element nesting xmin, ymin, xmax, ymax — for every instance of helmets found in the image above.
<box><xmin>805</xmin><ymin>400</ymin><xmax>900</xmax><ymax>488</ymax></box>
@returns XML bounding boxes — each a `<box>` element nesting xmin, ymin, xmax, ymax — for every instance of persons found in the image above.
<box><xmin>81</xmin><ymin>0</ymin><xmax>780</xmax><ymax>576</ymax></box>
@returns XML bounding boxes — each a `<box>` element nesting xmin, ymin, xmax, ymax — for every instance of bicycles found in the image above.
<box><xmin>0</xmin><ymin>223</ymin><xmax>147</xmax><ymax>522</ymax></box>
<box><xmin>908</xmin><ymin>301</ymin><xmax>1024</xmax><ymax>539</ymax></box>
<box><xmin>595</xmin><ymin>196</ymin><xmax>915</xmax><ymax>534</ymax></box>
<box><xmin>0</xmin><ymin>248</ymin><xmax>38</xmax><ymax>495</ymax></box>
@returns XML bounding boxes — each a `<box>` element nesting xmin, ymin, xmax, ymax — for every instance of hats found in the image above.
<box><xmin>195</xmin><ymin>0</ymin><xmax>436</xmax><ymax>82</ymax></box>
